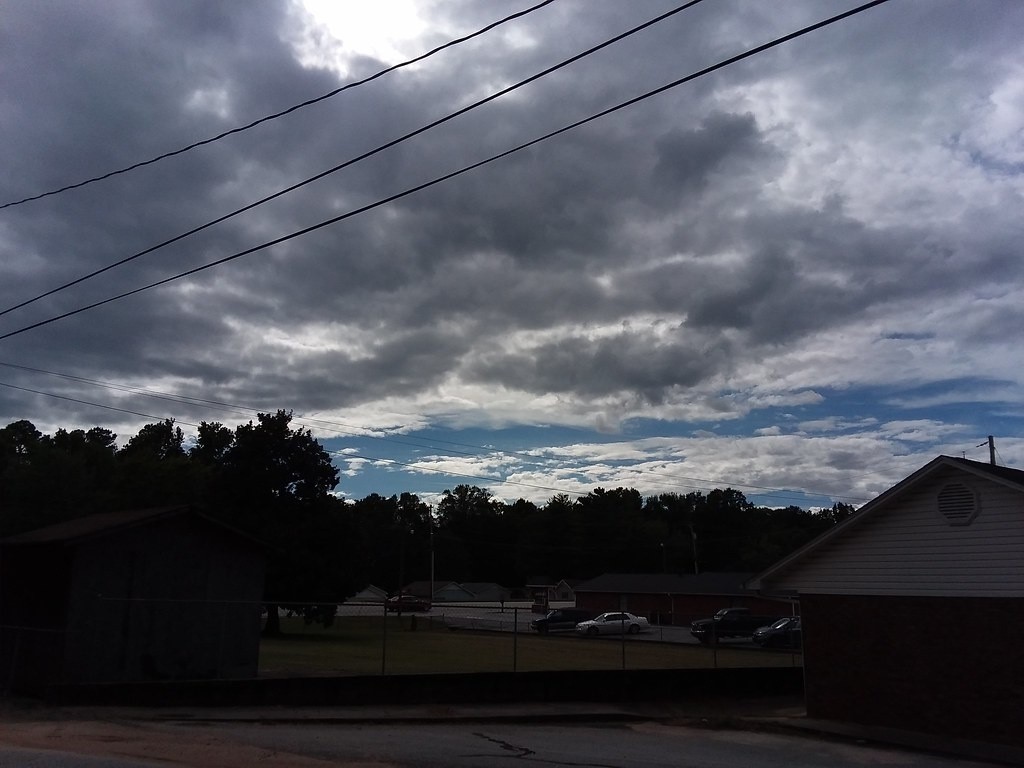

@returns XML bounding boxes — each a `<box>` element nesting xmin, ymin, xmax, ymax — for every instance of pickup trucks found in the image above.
<box><xmin>690</xmin><ymin>607</ymin><xmax>782</xmax><ymax>645</ymax></box>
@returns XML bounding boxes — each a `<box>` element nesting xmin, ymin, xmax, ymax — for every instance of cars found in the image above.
<box><xmin>751</xmin><ymin>616</ymin><xmax>802</xmax><ymax>652</ymax></box>
<box><xmin>530</xmin><ymin>607</ymin><xmax>593</xmax><ymax>633</ymax></box>
<box><xmin>576</xmin><ymin>611</ymin><xmax>651</xmax><ymax>635</ymax></box>
<box><xmin>385</xmin><ymin>595</ymin><xmax>432</xmax><ymax>612</ymax></box>
